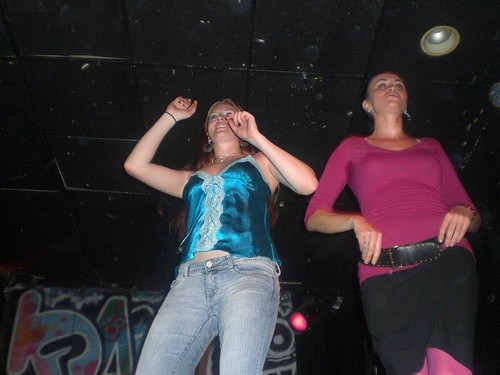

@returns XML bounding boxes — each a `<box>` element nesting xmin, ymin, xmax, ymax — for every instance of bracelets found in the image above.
<box><xmin>163</xmin><ymin>110</ymin><xmax>177</xmax><ymax>124</ymax></box>
<box><xmin>448</xmin><ymin>201</ymin><xmax>476</xmax><ymax>223</ymax></box>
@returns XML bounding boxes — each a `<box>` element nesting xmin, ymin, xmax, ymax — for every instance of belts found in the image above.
<box><xmin>355</xmin><ymin>231</ymin><xmax>447</xmax><ymax>268</ymax></box>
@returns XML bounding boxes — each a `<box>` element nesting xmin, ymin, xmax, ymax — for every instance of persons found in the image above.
<box><xmin>120</xmin><ymin>95</ymin><xmax>320</xmax><ymax>375</ymax></box>
<box><xmin>304</xmin><ymin>66</ymin><xmax>490</xmax><ymax>375</ymax></box>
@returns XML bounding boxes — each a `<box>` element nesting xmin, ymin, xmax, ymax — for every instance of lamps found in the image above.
<box><xmin>288</xmin><ymin>298</ymin><xmax>324</xmax><ymax>336</ymax></box>
